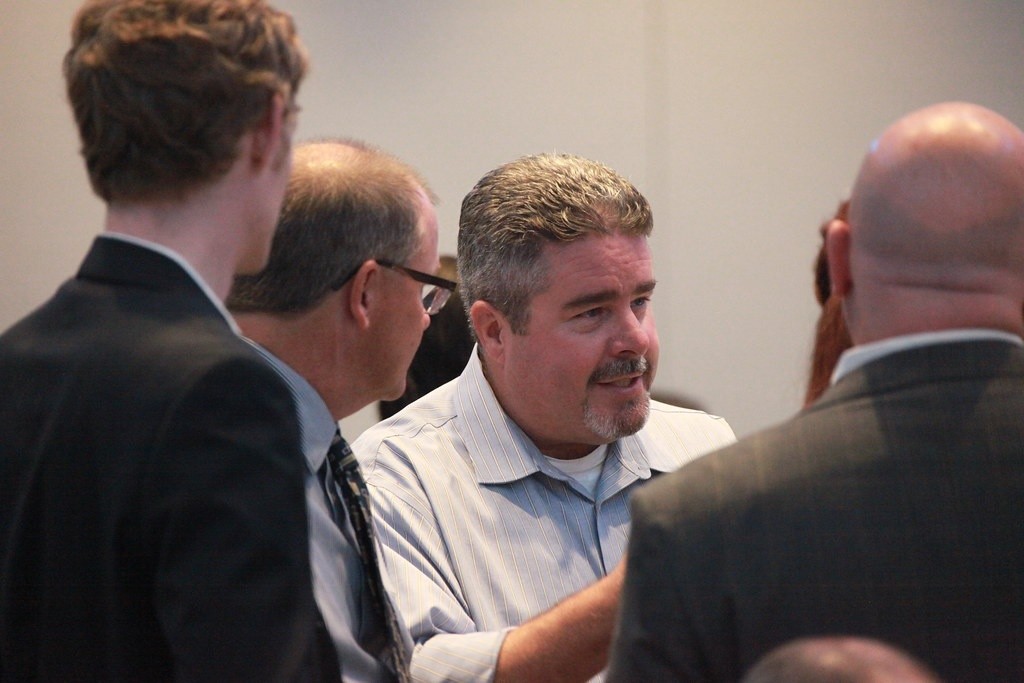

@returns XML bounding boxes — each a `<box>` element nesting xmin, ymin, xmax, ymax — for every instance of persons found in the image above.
<box><xmin>0</xmin><ymin>0</ymin><xmax>341</xmax><ymax>683</ymax></box>
<box><xmin>380</xmin><ymin>256</ymin><xmax>477</xmax><ymax>424</ymax></box>
<box><xmin>225</xmin><ymin>144</ymin><xmax>436</xmax><ymax>432</ymax></box>
<box><xmin>610</xmin><ymin>100</ymin><xmax>1024</xmax><ymax>683</ymax></box>
<box><xmin>343</xmin><ymin>154</ymin><xmax>739</xmax><ymax>683</ymax></box>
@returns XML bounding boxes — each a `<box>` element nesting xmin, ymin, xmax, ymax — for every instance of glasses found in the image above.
<box><xmin>333</xmin><ymin>258</ymin><xmax>456</xmax><ymax>314</ymax></box>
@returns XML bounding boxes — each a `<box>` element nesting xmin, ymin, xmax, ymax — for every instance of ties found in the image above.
<box><xmin>328</xmin><ymin>424</ymin><xmax>411</xmax><ymax>683</ymax></box>
